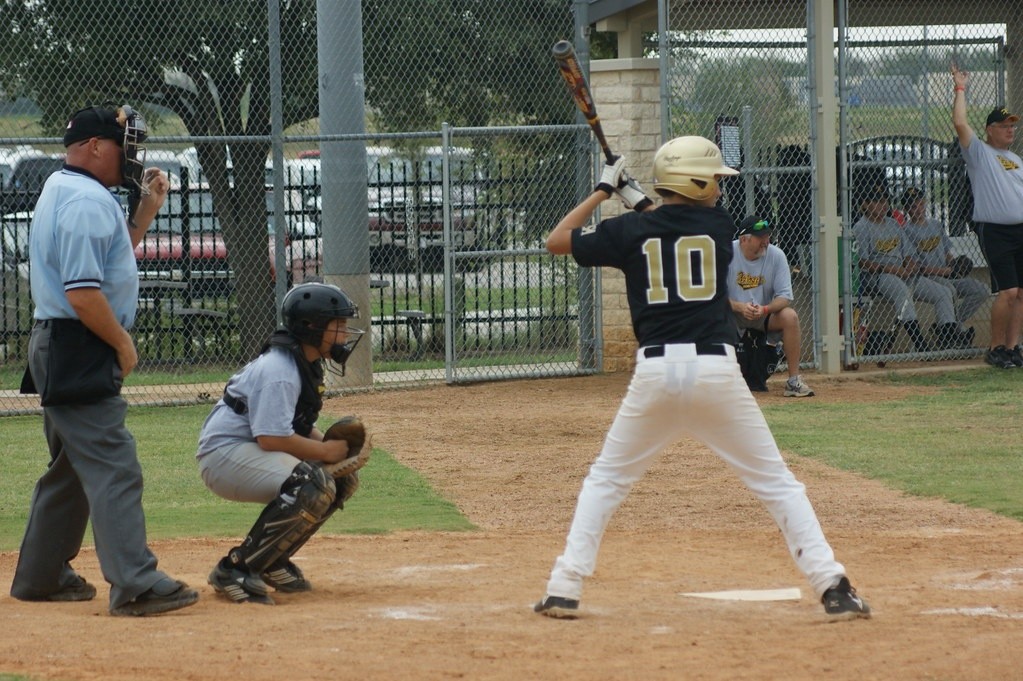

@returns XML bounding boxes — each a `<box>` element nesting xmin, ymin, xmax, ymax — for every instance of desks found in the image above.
<box><xmin>305</xmin><ymin>275</ymin><xmax>391</xmax><ymax>298</ymax></box>
<box><xmin>136</xmin><ymin>269</ymin><xmax>234</xmax><ymax>359</ymax></box>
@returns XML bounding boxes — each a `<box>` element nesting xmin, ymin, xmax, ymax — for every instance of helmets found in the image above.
<box><xmin>281</xmin><ymin>282</ymin><xmax>354</xmax><ymax>346</ymax></box>
<box><xmin>652</xmin><ymin>134</ymin><xmax>739</xmax><ymax>200</ymax></box>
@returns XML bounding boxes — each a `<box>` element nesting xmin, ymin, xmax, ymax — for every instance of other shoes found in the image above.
<box><xmin>962</xmin><ymin>327</ymin><xmax>975</xmax><ymax>347</ymax></box>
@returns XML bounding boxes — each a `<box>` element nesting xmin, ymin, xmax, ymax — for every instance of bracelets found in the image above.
<box><xmin>954</xmin><ymin>85</ymin><xmax>964</xmax><ymax>90</ymax></box>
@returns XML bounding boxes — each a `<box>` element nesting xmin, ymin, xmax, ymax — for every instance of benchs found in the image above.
<box><xmin>171</xmin><ymin>307</ymin><xmax>240</xmax><ymax>360</ymax></box>
<box><xmin>796</xmin><ymin>232</ymin><xmax>1001</xmax><ymax>353</ymax></box>
<box><xmin>397</xmin><ymin>310</ymin><xmax>429</xmax><ymax>362</ymax></box>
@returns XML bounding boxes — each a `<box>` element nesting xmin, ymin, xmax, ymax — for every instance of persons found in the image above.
<box><xmin>862</xmin><ymin>199</ymin><xmax>994</xmax><ymax>352</ymax></box>
<box><xmin>849</xmin><ymin>93</ymin><xmax>861</xmax><ymax>111</ymax></box>
<box><xmin>728</xmin><ymin>215</ymin><xmax>816</xmax><ymax>399</ymax></box>
<box><xmin>19</xmin><ymin>102</ymin><xmax>200</xmax><ymax>618</ymax></box>
<box><xmin>197</xmin><ymin>282</ymin><xmax>374</xmax><ymax>610</ymax></box>
<box><xmin>950</xmin><ymin>62</ymin><xmax>1023</xmax><ymax>370</ymax></box>
<box><xmin>533</xmin><ymin>135</ymin><xmax>872</xmax><ymax>623</ymax></box>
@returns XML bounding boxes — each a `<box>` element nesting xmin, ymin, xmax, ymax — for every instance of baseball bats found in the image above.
<box><xmin>551</xmin><ymin>37</ymin><xmax>614</xmax><ymax>164</ymax></box>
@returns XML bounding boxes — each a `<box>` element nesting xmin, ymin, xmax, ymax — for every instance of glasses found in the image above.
<box><xmin>740</xmin><ymin>221</ymin><xmax>769</xmax><ymax>235</ymax></box>
<box><xmin>79</xmin><ymin>136</ymin><xmax>133</xmax><ymax>151</ymax></box>
<box><xmin>990</xmin><ymin>124</ymin><xmax>1017</xmax><ymax>130</ymax></box>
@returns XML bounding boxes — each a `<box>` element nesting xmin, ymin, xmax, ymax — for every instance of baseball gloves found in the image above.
<box><xmin>321</xmin><ymin>415</ymin><xmax>373</xmax><ymax>478</ymax></box>
<box><xmin>950</xmin><ymin>255</ymin><xmax>973</xmax><ymax>279</ymax></box>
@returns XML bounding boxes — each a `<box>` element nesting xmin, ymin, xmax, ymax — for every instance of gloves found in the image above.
<box><xmin>594</xmin><ymin>154</ymin><xmax>626</xmax><ymax>197</ymax></box>
<box><xmin>614</xmin><ymin>173</ymin><xmax>653</xmax><ymax>212</ymax></box>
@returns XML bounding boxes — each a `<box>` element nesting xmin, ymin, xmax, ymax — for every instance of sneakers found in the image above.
<box><xmin>533</xmin><ymin>595</ymin><xmax>581</xmax><ymax>618</ymax></box>
<box><xmin>109</xmin><ymin>578</ymin><xmax>199</xmax><ymax>615</ymax></box>
<box><xmin>260</xmin><ymin>564</ymin><xmax>313</xmax><ymax>593</ymax></box>
<box><xmin>822</xmin><ymin>577</ymin><xmax>870</xmax><ymax>623</ymax></box>
<box><xmin>207</xmin><ymin>556</ymin><xmax>275</xmax><ymax>609</ymax></box>
<box><xmin>44</xmin><ymin>575</ymin><xmax>96</xmax><ymax>601</ymax></box>
<box><xmin>783</xmin><ymin>376</ymin><xmax>814</xmax><ymax>397</ymax></box>
<box><xmin>984</xmin><ymin>344</ymin><xmax>1023</xmax><ymax>369</ymax></box>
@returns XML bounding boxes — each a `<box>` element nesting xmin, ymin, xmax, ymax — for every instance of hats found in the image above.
<box><xmin>900</xmin><ymin>188</ymin><xmax>924</xmax><ymax>206</ymax></box>
<box><xmin>987</xmin><ymin>109</ymin><xmax>1019</xmax><ymax>125</ymax></box>
<box><xmin>740</xmin><ymin>215</ymin><xmax>771</xmax><ymax>236</ymax></box>
<box><xmin>64</xmin><ymin>105</ymin><xmax>147</xmax><ymax>147</ymax></box>
<box><xmin>864</xmin><ymin>184</ymin><xmax>889</xmax><ymax>200</ymax></box>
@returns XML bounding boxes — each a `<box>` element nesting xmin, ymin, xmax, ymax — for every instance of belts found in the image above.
<box><xmin>644</xmin><ymin>343</ymin><xmax>727</xmax><ymax>358</ymax></box>
<box><xmin>35</xmin><ymin>320</ymin><xmax>52</xmax><ymax>327</ymax></box>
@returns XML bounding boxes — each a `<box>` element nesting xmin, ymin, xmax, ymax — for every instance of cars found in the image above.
<box><xmin>857</xmin><ymin>145</ymin><xmax>924</xmax><ymax>195</ymax></box>
<box><xmin>0</xmin><ymin>144</ymin><xmax>507</xmax><ymax>295</ymax></box>
